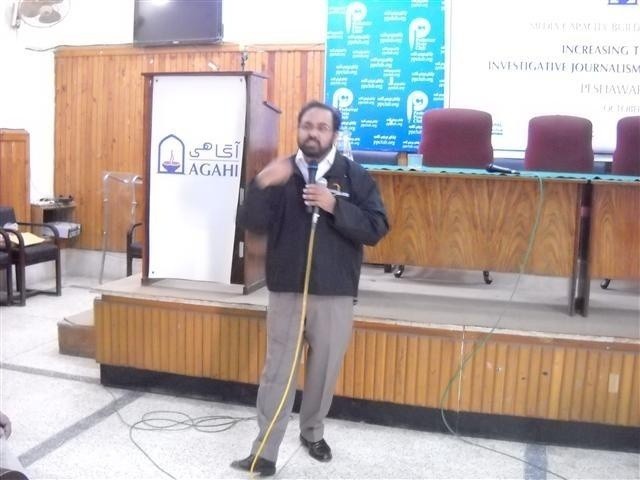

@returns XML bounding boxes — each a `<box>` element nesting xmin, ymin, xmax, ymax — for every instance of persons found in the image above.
<box><xmin>227</xmin><ymin>100</ymin><xmax>391</xmax><ymax>476</ymax></box>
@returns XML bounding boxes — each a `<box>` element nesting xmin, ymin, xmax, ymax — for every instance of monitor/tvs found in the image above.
<box><xmin>133</xmin><ymin>0</ymin><xmax>223</xmax><ymax>47</ymax></box>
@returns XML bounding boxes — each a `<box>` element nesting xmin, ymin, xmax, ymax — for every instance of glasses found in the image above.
<box><xmin>296</xmin><ymin>122</ymin><xmax>337</xmax><ymax>135</ymax></box>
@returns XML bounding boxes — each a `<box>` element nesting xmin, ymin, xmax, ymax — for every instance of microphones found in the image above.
<box><xmin>311</xmin><ymin>177</ymin><xmax>329</xmax><ymax>224</ymax></box>
<box><xmin>306</xmin><ymin>159</ymin><xmax>318</xmax><ymax>213</ymax></box>
<box><xmin>486</xmin><ymin>163</ymin><xmax>519</xmax><ymax>174</ymax></box>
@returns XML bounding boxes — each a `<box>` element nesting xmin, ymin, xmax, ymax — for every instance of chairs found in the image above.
<box><xmin>395</xmin><ymin>107</ymin><xmax>495</xmax><ymax>287</ymax></box>
<box><xmin>598</xmin><ymin>115</ymin><xmax>639</xmax><ymax>290</ymax></box>
<box><xmin>0</xmin><ymin>206</ymin><xmax>61</xmax><ymax>305</ymax></box>
<box><xmin>522</xmin><ymin>113</ymin><xmax>595</xmax><ymax>176</ymax></box>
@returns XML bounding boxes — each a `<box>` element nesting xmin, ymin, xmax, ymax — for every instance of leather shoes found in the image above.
<box><xmin>228</xmin><ymin>454</ymin><xmax>278</xmax><ymax>477</ymax></box>
<box><xmin>299</xmin><ymin>431</ymin><xmax>333</xmax><ymax>464</ymax></box>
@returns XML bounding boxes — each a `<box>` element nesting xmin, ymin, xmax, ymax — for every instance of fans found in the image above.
<box><xmin>10</xmin><ymin>0</ymin><xmax>70</xmax><ymax>33</ymax></box>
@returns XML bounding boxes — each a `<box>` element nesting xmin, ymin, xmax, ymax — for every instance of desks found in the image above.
<box><xmin>577</xmin><ymin>174</ymin><xmax>640</xmax><ymax>316</ymax></box>
<box><xmin>362</xmin><ymin>165</ymin><xmax>588</xmax><ymax>315</ymax></box>
